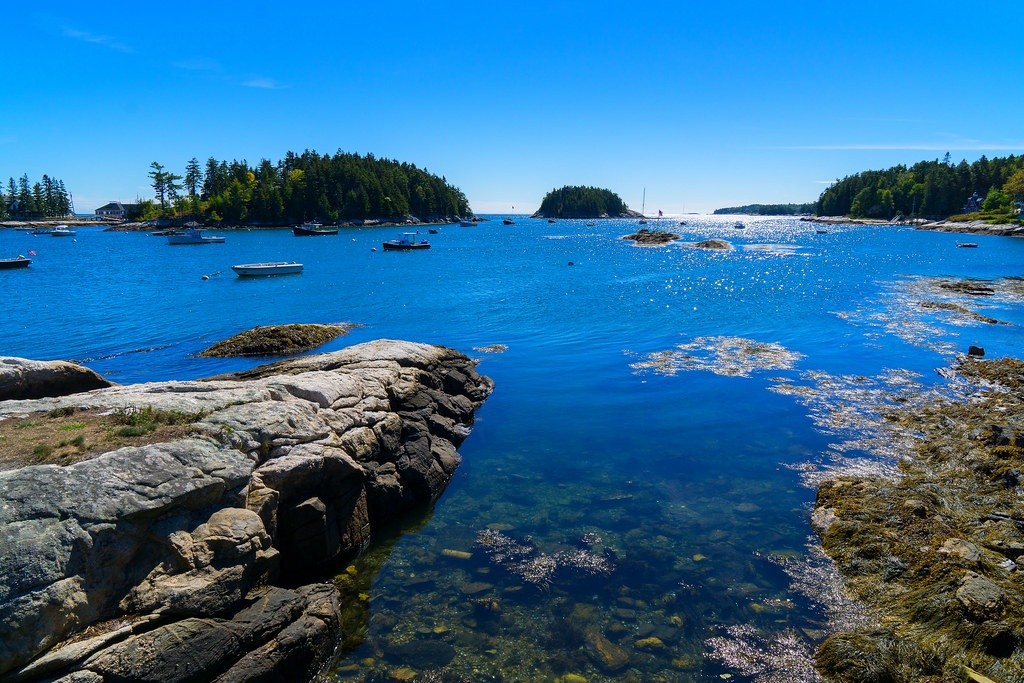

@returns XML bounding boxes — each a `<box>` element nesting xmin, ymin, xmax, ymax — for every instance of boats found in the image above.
<box><xmin>291</xmin><ymin>216</ymin><xmax>338</xmax><ymax>236</ymax></box>
<box><xmin>232</xmin><ymin>261</ymin><xmax>304</xmax><ymax>276</ymax></box>
<box><xmin>167</xmin><ymin>229</ymin><xmax>227</xmax><ymax>244</ymax></box>
<box><xmin>0</xmin><ymin>255</ymin><xmax>32</xmax><ymax>269</ymax></box>
<box><xmin>49</xmin><ymin>224</ymin><xmax>77</xmax><ymax>237</ymax></box>
<box><xmin>382</xmin><ymin>232</ymin><xmax>431</xmax><ymax>250</ymax></box>
